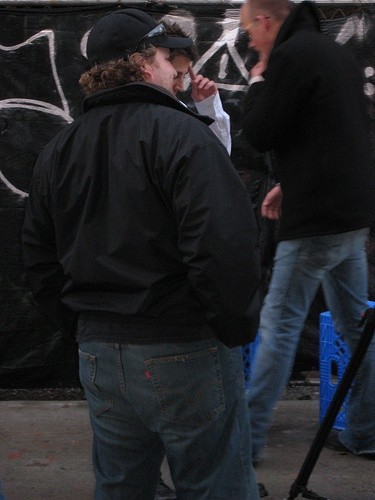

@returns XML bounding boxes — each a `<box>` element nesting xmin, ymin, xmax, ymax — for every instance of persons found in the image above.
<box><xmin>240</xmin><ymin>0</ymin><xmax>375</xmax><ymax>469</ymax></box>
<box><xmin>162</xmin><ymin>20</ymin><xmax>231</xmax><ymax>158</ymax></box>
<box><xmin>19</xmin><ymin>10</ymin><xmax>265</xmax><ymax>500</ymax></box>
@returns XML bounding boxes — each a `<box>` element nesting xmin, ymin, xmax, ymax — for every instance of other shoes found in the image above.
<box><xmin>325</xmin><ymin>430</ymin><xmax>375</xmax><ymax>460</ymax></box>
<box><xmin>258</xmin><ymin>483</ymin><xmax>267</xmax><ymax>496</ymax></box>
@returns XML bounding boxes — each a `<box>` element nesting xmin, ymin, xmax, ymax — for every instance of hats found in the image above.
<box><xmin>86</xmin><ymin>9</ymin><xmax>193</xmax><ymax>68</ymax></box>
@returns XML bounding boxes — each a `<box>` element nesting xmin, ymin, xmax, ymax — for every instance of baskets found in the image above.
<box><xmin>318</xmin><ymin>300</ymin><xmax>375</xmax><ymax>430</ymax></box>
<box><xmin>241</xmin><ymin>329</ymin><xmax>262</xmax><ymax>390</ymax></box>
